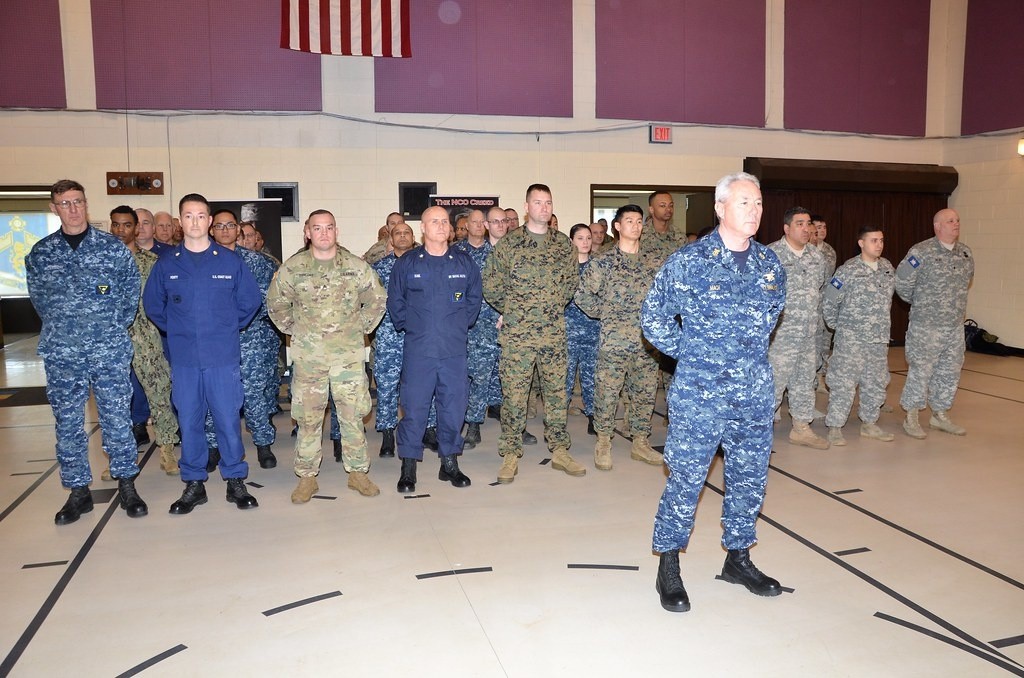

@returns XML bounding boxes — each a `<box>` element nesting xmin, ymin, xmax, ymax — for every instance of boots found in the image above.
<box><xmin>438</xmin><ymin>454</ymin><xmax>471</xmax><ymax>487</ymax></box>
<box><xmin>160</xmin><ymin>443</ymin><xmax>181</xmax><ymax>475</ymax></box>
<box><xmin>55</xmin><ymin>485</ymin><xmax>95</xmax><ymax>524</ymax></box>
<box><xmin>169</xmin><ymin>481</ymin><xmax>208</xmax><ymax>515</ymax></box>
<box><xmin>656</xmin><ymin>551</ymin><xmax>690</xmax><ymax>612</ymax></box>
<box><xmin>205</xmin><ymin>403</ymin><xmax>536</xmax><ymax>474</ymax></box>
<box><xmin>226</xmin><ymin>478</ymin><xmax>258</xmax><ymax>509</ymax></box>
<box><xmin>723</xmin><ymin>549</ymin><xmax>783</xmax><ymax>596</ymax></box>
<box><xmin>101</xmin><ymin>450</ymin><xmax>113</xmax><ymax>481</ymax></box>
<box><xmin>118</xmin><ymin>478</ymin><xmax>148</xmax><ymax>518</ymax></box>
<box><xmin>397</xmin><ymin>457</ymin><xmax>417</xmax><ymax>492</ymax></box>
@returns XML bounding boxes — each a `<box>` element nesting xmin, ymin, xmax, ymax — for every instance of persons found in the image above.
<box><xmin>266</xmin><ymin>184</ymin><xmax>580</xmax><ymax>504</ymax></box>
<box><xmin>24</xmin><ymin>179</ymin><xmax>149</xmax><ymax>526</ymax></box>
<box><xmin>564</xmin><ymin>190</ymin><xmax>715</xmax><ymax>471</ymax></box>
<box><xmin>101</xmin><ymin>193</ymin><xmax>281</xmax><ymax>515</ymax></box>
<box><xmin>765</xmin><ymin>206</ymin><xmax>837</xmax><ymax>450</ymax></box>
<box><xmin>641</xmin><ymin>172</ymin><xmax>787</xmax><ymax>612</ymax></box>
<box><xmin>822</xmin><ymin>226</ymin><xmax>897</xmax><ymax>446</ymax></box>
<box><xmin>240</xmin><ymin>203</ymin><xmax>265</xmax><ymax>230</ymax></box>
<box><xmin>895</xmin><ymin>209</ymin><xmax>975</xmax><ymax>440</ymax></box>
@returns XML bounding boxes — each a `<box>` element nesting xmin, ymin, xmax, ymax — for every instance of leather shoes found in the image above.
<box><xmin>132</xmin><ymin>426</ymin><xmax>150</xmax><ymax>449</ymax></box>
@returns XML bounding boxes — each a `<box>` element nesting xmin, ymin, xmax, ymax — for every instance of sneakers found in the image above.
<box><xmin>902</xmin><ymin>408</ymin><xmax>927</xmax><ymax>439</ymax></box>
<box><xmin>631</xmin><ymin>436</ymin><xmax>665</xmax><ymax>465</ymax></box>
<box><xmin>552</xmin><ymin>447</ymin><xmax>587</xmax><ymax>476</ymax></box>
<box><xmin>496</xmin><ymin>453</ymin><xmax>519</xmax><ymax>483</ymax></box>
<box><xmin>789</xmin><ymin>419</ymin><xmax>830</xmax><ymax>449</ymax></box>
<box><xmin>527</xmin><ymin>393</ymin><xmax>894</xmax><ymax>436</ymax></box>
<box><xmin>861</xmin><ymin>423</ymin><xmax>895</xmax><ymax>442</ymax></box>
<box><xmin>347</xmin><ymin>471</ymin><xmax>380</xmax><ymax>497</ymax></box>
<box><xmin>827</xmin><ymin>427</ymin><xmax>847</xmax><ymax>446</ymax></box>
<box><xmin>594</xmin><ymin>434</ymin><xmax>612</xmax><ymax>470</ymax></box>
<box><xmin>929</xmin><ymin>411</ymin><xmax>967</xmax><ymax>436</ymax></box>
<box><xmin>291</xmin><ymin>476</ymin><xmax>319</xmax><ymax>503</ymax></box>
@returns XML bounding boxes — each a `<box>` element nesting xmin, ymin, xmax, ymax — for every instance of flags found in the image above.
<box><xmin>279</xmin><ymin>0</ymin><xmax>412</xmax><ymax>59</ymax></box>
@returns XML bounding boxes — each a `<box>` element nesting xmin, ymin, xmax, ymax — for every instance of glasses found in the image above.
<box><xmin>487</xmin><ymin>218</ymin><xmax>508</xmax><ymax>227</ymax></box>
<box><xmin>52</xmin><ymin>199</ymin><xmax>86</xmax><ymax>209</ymax></box>
<box><xmin>212</xmin><ymin>223</ymin><xmax>238</xmax><ymax>230</ymax></box>
